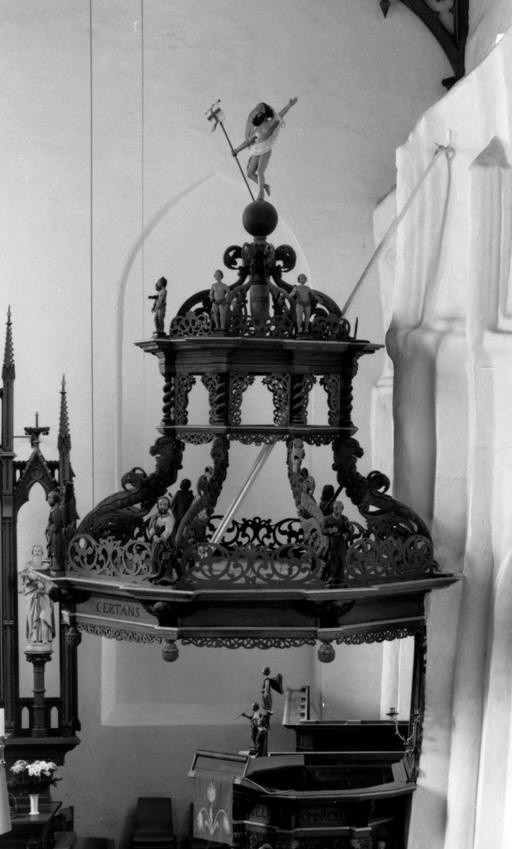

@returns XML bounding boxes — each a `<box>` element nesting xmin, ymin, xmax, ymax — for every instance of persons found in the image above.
<box><xmin>15</xmin><ymin>544</ymin><xmax>55</xmax><ymax>647</ymax></box>
<box><xmin>248</xmin><ymin>717</ymin><xmax>270</xmax><ymax>757</ymax></box>
<box><xmin>147</xmin><ymin>496</ymin><xmax>179</xmax><ymax>586</ymax></box>
<box><xmin>262</xmin><ymin>664</ymin><xmax>286</xmax><ymax>715</ymax></box>
<box><xmin>232</xmin><ymin>94</ymin><xmax>300</xmax><ymax>201</ymax></box>
<box><xmin>208</xmin><ymin>271</ymin><xmax>230</xmax><ymax>330</ymax></box>
<box><xmin>316</xmin><ymin>501</ymin><xmax>352</xmax><ymax>586</ymax></box>
<box><xmin>147</xmin><ymin>278</ymin><xmax>168</xmax><ymax>334</ymax></box>
<box><xmin>288</xmin><ymin>272</ymin><xmax>321</xmax><ymax>331</ymax></box>
<box><xmin>240</xmin><ymin>701</ymin><xmax>262</xmax><ymax>751</ymax></box>
